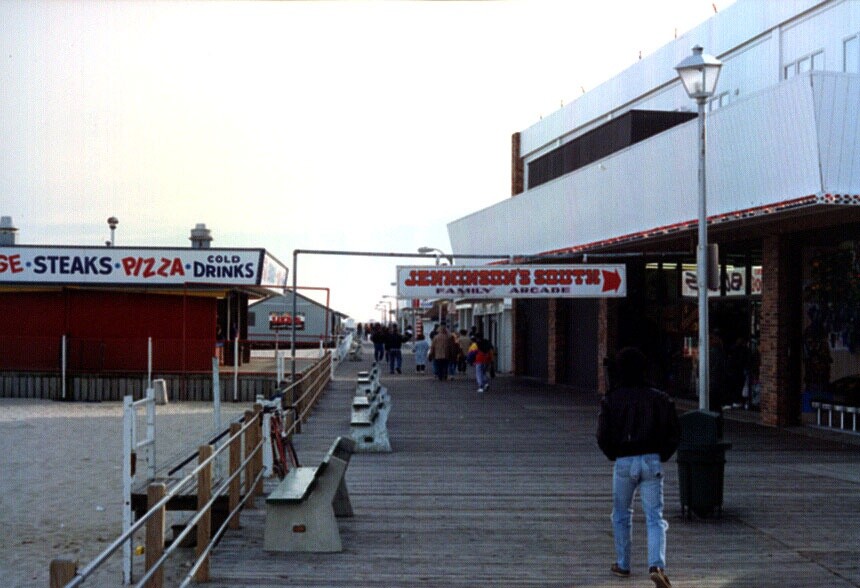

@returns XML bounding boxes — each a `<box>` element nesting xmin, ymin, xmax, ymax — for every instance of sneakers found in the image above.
<box><xmin>649</xmin><ymin>566</ymin><xmax>671</xmax><ymax>588</ymax></box>
<box><xmin>611</xmin><ymin>564</ymin><xmax>630</xmax><ymax>576</ymax></box>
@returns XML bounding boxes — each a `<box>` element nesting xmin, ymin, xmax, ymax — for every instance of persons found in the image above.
<box><xmin>428</xmin><ymin>322</ymin><xmax>497</xmax><ymax>393</ymax></box>
<box><xmin>596</xmin><ymin>355</ymin><xmax>682</xmax><ymax>587</ymax></box>
<box><xmin>410</xmin><ymin>333</ymin><xmax>429</xmax><ymax>376</ymax></box>
<box><xmin>358</xmin><ymin>320</ymin><xmax>402</xmax><ymax>375</ymax></box>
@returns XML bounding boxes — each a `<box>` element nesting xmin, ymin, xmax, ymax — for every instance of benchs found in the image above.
<box><xmin>349</xmin><ymin>334</ymin><xmax>365</xmax><ymax>362</ymax></box>
<box><xmin>356</xmin><ymin>368</ymin><xmax>381</xmax><ymax>396</ymax></box>
<box><xmin>263</xmin><ymin>434</ymin><xmax>356</xmax><ymax>553</ymax></box>
<box><xmin>357</xmin><ymin>361</ymin><xmax>380</xmax><ymax>378</ymax></box>
<box><xmin>350</xmin><ymin>383</ymin><xmax>389</xmax><ymax>414</ymax></box>
<box><xmin>349</xmin><ymin>392</ymin><xmax>394</xmax><ymax>454</ymax></box>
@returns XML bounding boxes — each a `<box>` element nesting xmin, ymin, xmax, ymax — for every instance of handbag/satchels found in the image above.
<box><xmin>656</xmin><ymin>395</ymin><xmax>680</xmax><ymax>462</ymax></box>
<box><xmin>429</xmin><ymin>352</ymin><xmax>435</xmax><ymax>362</ymax></box>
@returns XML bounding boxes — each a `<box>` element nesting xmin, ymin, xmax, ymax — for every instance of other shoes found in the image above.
<box><xmin>396</xmin><ymin>368</ymin><xmax>402</xmax><ymax>374</ymax></box>
<box><xmin>478</xmin><ymin>388</ymin><xmax>483</xmax><ymax>393</ymax></box>
<box><xmin>484</xmin><ymin>383</ymin><xmax>489</xmax><ymax>392</ymax></box>
<box><xmin>421</xmin><ymin>371</ymin><xmax>425</xmax><ymax>375</ymax></box>
